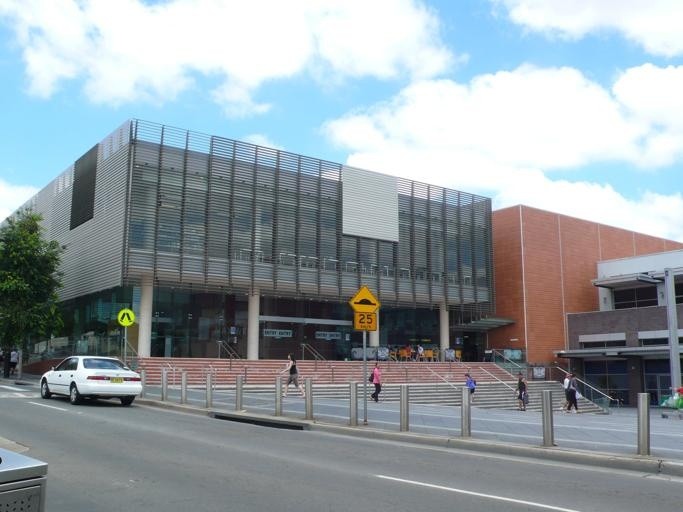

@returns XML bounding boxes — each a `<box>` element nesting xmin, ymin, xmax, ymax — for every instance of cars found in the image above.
<box><xmin>40</xmin><ymin>356</ymin><xmax>142</xmax><ymax>405</ymax></box>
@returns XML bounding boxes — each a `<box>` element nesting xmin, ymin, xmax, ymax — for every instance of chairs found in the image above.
<box><xmin>389</xmin><ymin>347</ymin><xmax>440</xmax><ymax>361</ymax></box>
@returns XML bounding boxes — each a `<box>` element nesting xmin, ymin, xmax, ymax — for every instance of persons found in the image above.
<box><xmin>514</xmin><ymin>371</ymin><xmax>528</xmax><ymax>411</ymax></box>
<box><xmin>559</xmin><ymin>373</ymin><xmax>577</xmax><ymax>414</ymax></box>
<box><xmin>566</xmin><ymin>374</ymin><xmax>582</xmax><ymax>412</ymax></box>
<box><xmin>372</xmin><ymin>344</ymin><xmax>423</xmax><ymax>362</ymax></box>
<box><xmin>280</xmin><ymin>352</ymin><xmax>305</xmax><ymax>399</ymax></box>
<box><xmin>464</xmin><ymin>373</ymin><xmax>475</xmax><ymax>402</ymax></box>
<box><xmin>369</xmin><ymin>363</ymin><xmax>381</xmax><ymax>404</ymax></box>
<box><xmin>9</xmin><ymin>349</ymin><xmax>19</xmax><ymax>374</ymax></box>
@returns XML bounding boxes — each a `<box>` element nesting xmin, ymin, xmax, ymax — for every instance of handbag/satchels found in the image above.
<box><xmin>523</xmin><ymin>393</ymin><xmax>529</xmax><ymax>405</ymax></box>
<box><xmin>369</xmin><ymin>368</ymin><xmax>375</xmax><ymax>382</ymax></box>
